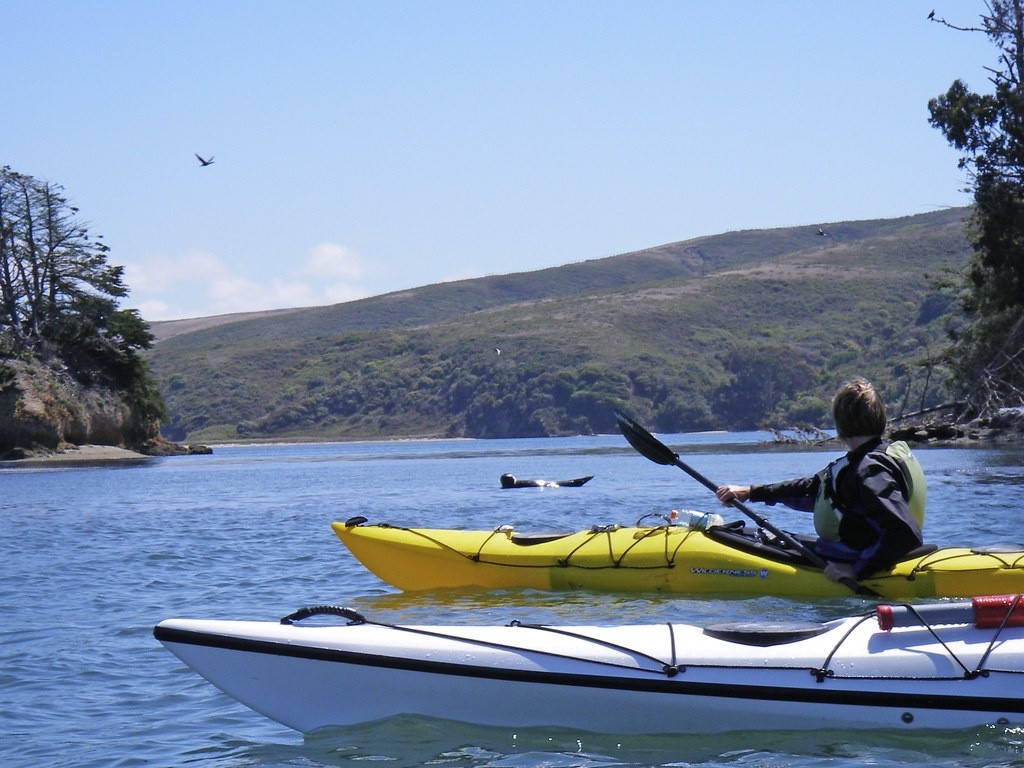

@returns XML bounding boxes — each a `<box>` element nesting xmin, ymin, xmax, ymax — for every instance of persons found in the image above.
<box><xmin>716</xmin><ymin>378</ymin><xmax>927</xmax><ymax>582</ymax></box>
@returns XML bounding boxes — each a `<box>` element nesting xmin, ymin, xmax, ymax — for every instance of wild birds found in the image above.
<box><xmin>195</xmin><ymin>154</ymin><xmax>215</xmax><ymax>167</ymax></box>
<box><xmin>493</xmin><ymin>348</ymin><xmax>503</xmax><ymax>355</ymax></box>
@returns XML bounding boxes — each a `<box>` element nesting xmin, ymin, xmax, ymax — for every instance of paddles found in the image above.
<box><xmin>605</xmin><ymin>405</ymin><xmax>883</xmax><ymax>599</ymax></box>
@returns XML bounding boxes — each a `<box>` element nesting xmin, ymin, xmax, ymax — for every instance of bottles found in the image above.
<box><xmin>670</xmin><ymin>508</ymin><xmax>724</xmax><ymax>529</ymax></box>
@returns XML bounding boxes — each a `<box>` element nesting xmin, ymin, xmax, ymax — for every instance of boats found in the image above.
<box><xmin>500</xmin><ymin>474</ymin><xmax>593</xmax><ymax>490</ymax></box>
<box><xmin>152</xmin><ymin>594</ymin><xmax>1024</xmax><ymax>730</ymax></box>
<box><xmin>330</xmin><ymin>510</ymin><xmax>1024</xmax><ymax>609</ymax></box>
